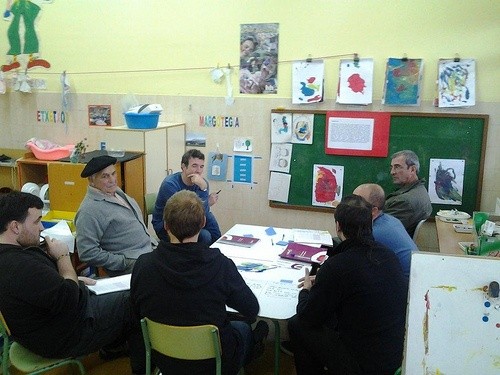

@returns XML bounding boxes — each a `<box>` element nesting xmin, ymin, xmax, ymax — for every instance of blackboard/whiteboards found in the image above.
<box><xmin>269</xmin><ymin>108</ymin><xmax>490</xmax><ymax>222</ymax></box>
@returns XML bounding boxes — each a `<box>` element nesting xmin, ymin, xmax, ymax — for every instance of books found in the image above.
<box><xmin>216</xmin><ymin>234</ymin><xmax>260</xmax><ymax>249</ymax></box>
<box><xmin>279</xmin><ymin>229</ymin><xmax>333</xmax><ymax>264</ymax></box>
<box><xmin>84</xmin><ymin>273</ymin><xmax>133</xmax><ymax>296</ymax></box>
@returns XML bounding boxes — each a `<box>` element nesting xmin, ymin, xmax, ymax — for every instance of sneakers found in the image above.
<box><xmin>280</xmin><ymin>340</ymin><xmax>294</xmax><ymax>356</ymax></box>
<box><xmin>252</xmin><ymin>320</ymin><xmax>268</xmax><ymax>361</ymax></box>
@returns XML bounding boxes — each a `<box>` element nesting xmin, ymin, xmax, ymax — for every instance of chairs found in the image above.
<box><xmin>140</xmin><ymin>317</ymin><xmax>222</xmax><ymax>375</ymax></box>
<box><xmin>0</xmin><ymin>310</ymin><xmax>85</xmax><ymax>375</ymax></box>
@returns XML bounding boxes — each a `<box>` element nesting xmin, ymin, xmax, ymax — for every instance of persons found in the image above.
<box><xmin>240</xmin><ymin>35</ymin><xmax>278</xmax><ymax>94</ymax></box>
<box><xmin>129</xmin><ymin>190</ymin><xmax>270</xmax><ymax>375</ymax></box>
<box><xmin>151</xmin><ymin>149</ymin><xmax>222</xmax><ymax>247</ymax></box>
<box><xmin>74</xmin><ymin>155</ymin><xmax>160</xmax><ymax>276</ymax></box>
<box><xmin>353</xmin><ymin>183</ymin><xmax>420</xmax><ymax>289</ymax></box>
<box><xmin>280</xmin><ymin>194</ymin><xmax>407</xmax><ymax>375</ymax></box>
<box><xmin>0</xmin><ymin>187</ymin><xmax>131</xmax><ymax>361</ymax></box>
<box><xmin>381</xmin><ymin>150</ymin><xmax>433</xmax><ymax>239</ymax></box>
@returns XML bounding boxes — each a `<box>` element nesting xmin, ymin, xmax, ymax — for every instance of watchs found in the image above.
<box><xmin>199</xmin><ymin>187</ymin><xmax>208</xmax><ymax>192</ymax></box>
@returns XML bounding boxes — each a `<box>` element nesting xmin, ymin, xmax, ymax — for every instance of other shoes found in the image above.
<box><xmin>99</xmin><ymin>341</ymin><xmax>130</xmax><ymax>361</ymax></box>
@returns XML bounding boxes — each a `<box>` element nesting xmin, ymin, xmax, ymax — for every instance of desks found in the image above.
<box><xmin>209</xmin><ymin>224</ymin><xmax>333</xmax><ymax>375</ymax></box>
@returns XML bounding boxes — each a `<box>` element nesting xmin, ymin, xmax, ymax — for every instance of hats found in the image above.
<box><xmin>81</xmin><ymin>155</ymin><xmax>117</xmax><ymax>178</ymax></box>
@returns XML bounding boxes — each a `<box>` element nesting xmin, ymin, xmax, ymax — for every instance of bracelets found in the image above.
<box><xmin>55</xmin><ymin>249</ymin><xmax>70</xmax><ymax>265</ymax></box>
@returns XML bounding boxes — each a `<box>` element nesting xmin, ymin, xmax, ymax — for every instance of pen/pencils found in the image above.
<box><xmin>216</xmin><ymin>190</ymin><xmax>221</xmax><ymax>194</ymax></box>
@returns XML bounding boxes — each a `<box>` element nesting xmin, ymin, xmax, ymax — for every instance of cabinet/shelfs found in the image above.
<box><xmin>16</xmin><ymin>149</ymin><xmax>146</xmax><ymax>220</ymax></box>
<box><xmin>106</xmin><ymin>122</ymin><xmax>186</xmax><ymax>214</ymax></box>
